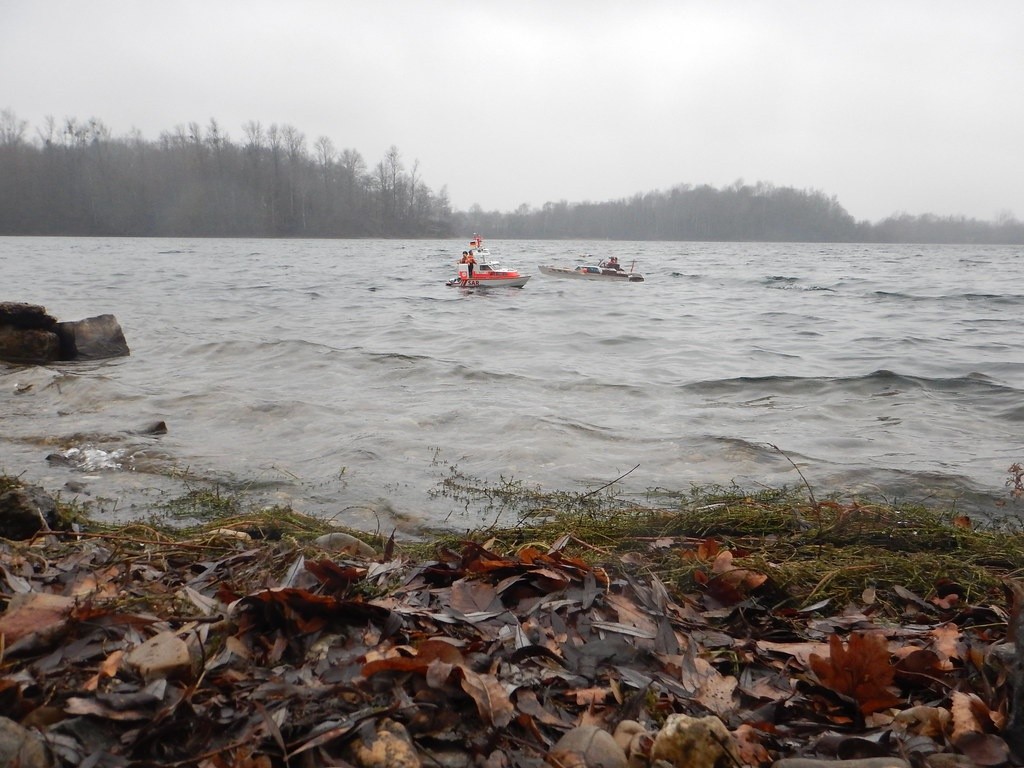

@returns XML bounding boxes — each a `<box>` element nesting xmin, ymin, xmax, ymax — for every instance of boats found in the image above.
<box><xmin>444</xmin><ymin>233</ymin><xmax>531</xmax><ymax>288</ymax></box>
<box><xmin>536</xmin><ymin>256</ymin><xmax>646</xmax><ymax>284</ymax></box>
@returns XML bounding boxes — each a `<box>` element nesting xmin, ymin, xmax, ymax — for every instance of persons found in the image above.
<box><xmin>460</xmin><ymin>249</ymin><xmax>477</xmax><ymax>278</ymax></box>
<box><xmin>608</xmin><ymin>256</ymin><xmax>617</xmax><ymax>268</ymax></box>
